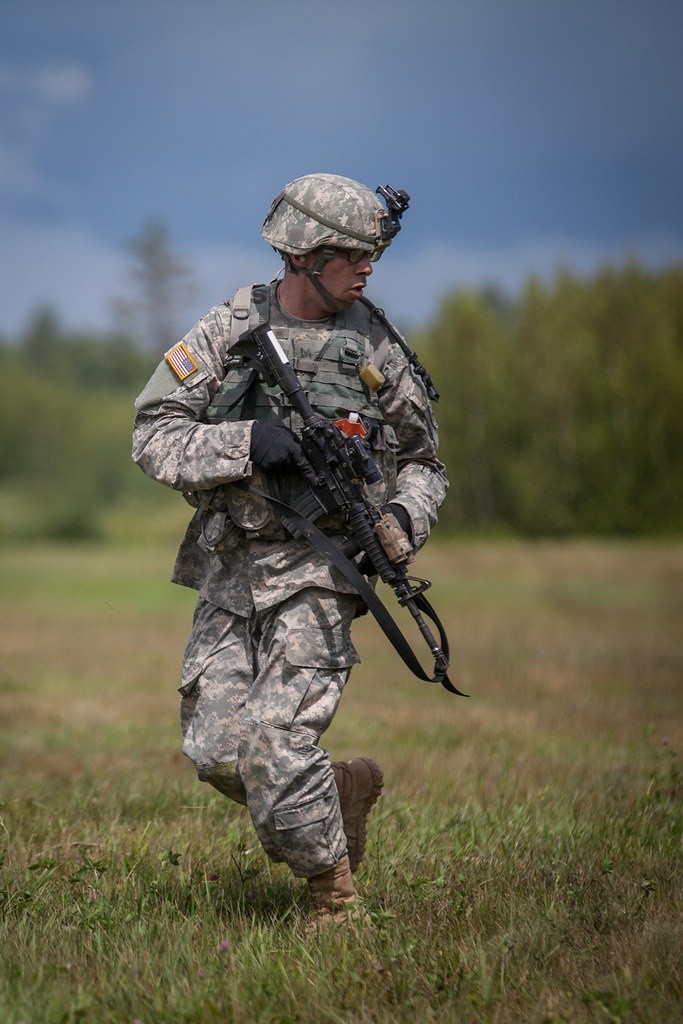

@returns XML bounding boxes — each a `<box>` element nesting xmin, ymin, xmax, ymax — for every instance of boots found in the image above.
<box><xmin>294</xmin><ymin>854</ymin><xmax>368</xmax><ymax>942</ymax></box>
<box><xmin>326</xmin><ymin>755</ymin><xmax>384</xmax><ymax>874</ymax></box>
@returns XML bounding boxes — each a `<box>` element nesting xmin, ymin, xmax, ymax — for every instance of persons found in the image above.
<box><xmin>132</xmin><ymin>172</ymin><xmax>451</xmax><ymax>943</ymax></box>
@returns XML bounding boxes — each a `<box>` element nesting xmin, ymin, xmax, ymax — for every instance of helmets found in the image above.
<box><xmin>261</xmin><ymin>173</ymin><xmax>392</xmax><ymax>254</ymax></box>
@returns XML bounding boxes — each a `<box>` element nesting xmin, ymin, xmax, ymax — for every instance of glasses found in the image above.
<box><xmin>326</xmin><ymin>243</ymin><xmax>383</xmax><ymax>264</ymax></box>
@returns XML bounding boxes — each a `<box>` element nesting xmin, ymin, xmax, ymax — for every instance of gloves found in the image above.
<box><xmin>250</xmin><ymin>421</ymin><xmax>319</xmax><ymax>487</ymax></box>
<box><xmin>357</xmin><ymin>503</ymin><xmax>412</xmax><ymax>577</ymax></box>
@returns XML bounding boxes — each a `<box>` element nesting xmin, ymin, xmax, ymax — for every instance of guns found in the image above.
<box><xmin>227</xmin><ymin>321</ymin><xmax>451</xmax><ymax>676</ymax></box>
